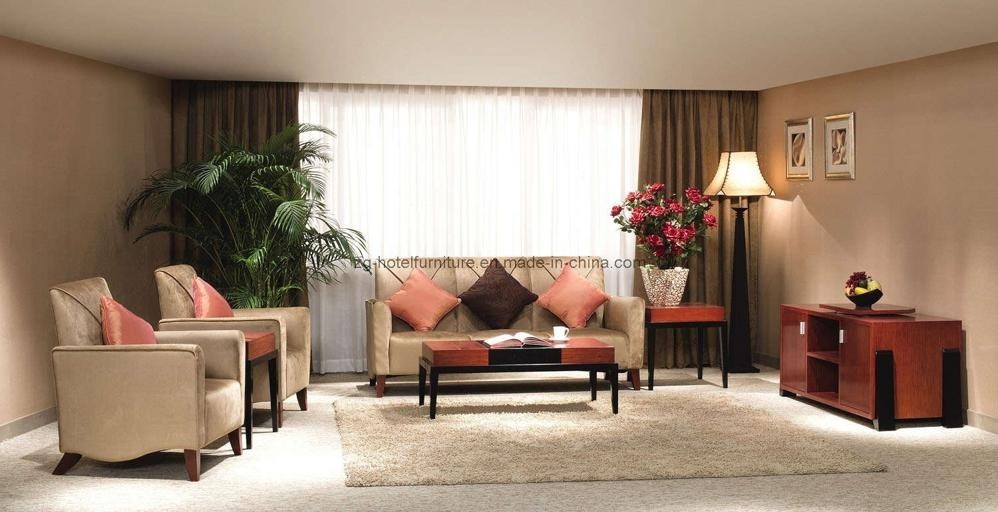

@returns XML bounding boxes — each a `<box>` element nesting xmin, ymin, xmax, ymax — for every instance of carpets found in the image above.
<box><xmin>330</xmin><ymin>387</ymin><xmax>891</xmax><ymax>494</ymax></box>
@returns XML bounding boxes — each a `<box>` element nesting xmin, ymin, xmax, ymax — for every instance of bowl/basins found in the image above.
<box><xmin>843</xmin><ymin>290</ymin><xmax>882</xmax><ymax>311</ymax></box>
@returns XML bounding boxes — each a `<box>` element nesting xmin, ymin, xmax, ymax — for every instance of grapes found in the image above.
<box><xmin>845</xmin><ymin>271</ymin><xmax>865</xmax><ymax>294</ymax></box>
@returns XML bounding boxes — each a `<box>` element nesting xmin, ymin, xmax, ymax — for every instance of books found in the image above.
<box><xmin>480</xmin><ymin>332</ymin><xmax>552</xmax><ymax>350</ymax></box>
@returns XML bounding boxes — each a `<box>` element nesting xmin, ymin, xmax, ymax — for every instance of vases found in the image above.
<box><xmin>638</xmin><ymin>259</ymin><xmax>692</xmax><ymax>310</ymax></box>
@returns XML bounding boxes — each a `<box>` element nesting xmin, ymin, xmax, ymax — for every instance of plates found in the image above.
<box><xmin>549</xmin><ymin>337</ymin><xmax>570</xmax><ymax>342</ymax></box>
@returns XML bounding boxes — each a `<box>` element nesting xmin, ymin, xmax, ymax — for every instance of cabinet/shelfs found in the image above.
<box><xmin>776</xmin><ymin>301</ymin><xmax>965</xmax><ymax>434</ymax></box>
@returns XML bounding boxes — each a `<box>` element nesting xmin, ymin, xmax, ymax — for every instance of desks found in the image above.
<box><xmin>414</xmin><ymin>335</ymin><xmax>623</xmax><ymax>421</ymax></box>
<box><xmin>642</xmin><ymin>296</ymin><xmax>730</xmax><ymax>393</ymax></box>
<box><xmin>235</xmin><ymin>331</ymin><xmax>282</xmax><ymax>451</ymax></box>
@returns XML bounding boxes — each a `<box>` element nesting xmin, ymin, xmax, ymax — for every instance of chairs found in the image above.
<box><xmin>44</xmin><ymin>270</ymin><xmax>249</xmax><ymax>485</ymax></box>
<box><xmin>152</xmin><ymin>260</ymin><xmax>315</xmax><ymax>430</ymax></box>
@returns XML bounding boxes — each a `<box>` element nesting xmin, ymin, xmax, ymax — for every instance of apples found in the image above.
<box><xmin>869</xmin><ymin>278</ymin><xmax>882</xmax><ymax>292</ymax></box>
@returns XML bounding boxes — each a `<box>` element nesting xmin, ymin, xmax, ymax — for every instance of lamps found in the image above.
<box><xmin>700</xmin><ymin>147</ymin><xmax>780</xmax><ymax>377</ymax></box>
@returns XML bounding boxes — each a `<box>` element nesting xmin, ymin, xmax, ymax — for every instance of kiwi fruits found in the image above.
<box><xmin>859</xmin><ymin>280</ymin><xmax>867</xmax><ymax>288</ymax></box>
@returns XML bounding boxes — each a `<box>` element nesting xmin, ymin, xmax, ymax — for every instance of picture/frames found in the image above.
<box><xmin>821</xmin><ymin>112</ymin><xmax>858</xmax><ymax>183</ymax></box>
<box><xmin>782</xmin><ymin>114</ymin><xmax>816</xmax><ymax>181</ymax></box>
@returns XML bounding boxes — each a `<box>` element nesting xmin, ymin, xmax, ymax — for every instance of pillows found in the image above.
<box><xmin>191</xmin><ymin>275</ymin><xmax>236</xmax><ymax>320</ymax></box>
<box><xmin>385</xmin><ymin>266</ymin><xmax>462</xmax><ymax>336</ymax></box>
<box><xmin>457</xmin><ymin>255</ymin><xmax>540</xmax><ymax>331</ymax></box>
<box><xmin>98</xmin><ymin>293</ymin><xmax>160</xmax><ymax>347</ymax></box>
<box><xmin>536</xmin><ymin>262</ymin><xmax>613</xmax><ymax>332</ymax></box>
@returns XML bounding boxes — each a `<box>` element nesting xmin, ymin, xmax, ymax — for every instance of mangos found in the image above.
<box><xmin>855</xmin><ymin>287</ymin><xmax>867</xmax><ymax>295</ymax></box>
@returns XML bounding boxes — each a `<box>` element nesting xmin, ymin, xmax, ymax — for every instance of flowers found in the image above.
<box><xmin>608</xmin><ymin>182</ymin><xmax>720</xmax><ymax>268</ymax></box>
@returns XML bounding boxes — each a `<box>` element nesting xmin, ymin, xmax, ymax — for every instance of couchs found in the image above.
<box><xmin>361</xmin><ymin>252</ymin><xmax>647</xmax><ymax>399</ymax></box>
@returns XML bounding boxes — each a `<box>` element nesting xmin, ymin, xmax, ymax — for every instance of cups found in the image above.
<box><xmin>553</xmin><ymin>325</ymin><xmax>569</xmax><ymax>338</ymax></box>
